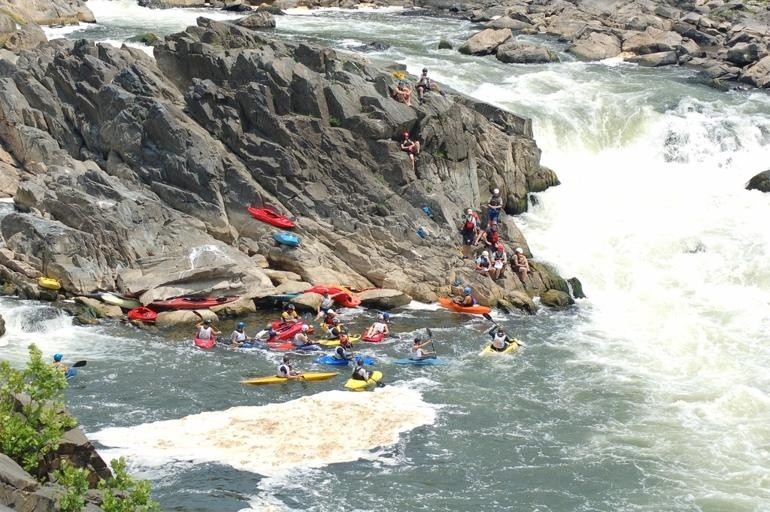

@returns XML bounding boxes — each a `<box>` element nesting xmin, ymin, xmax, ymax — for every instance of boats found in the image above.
<box><xmin>128</xmin><ymin>298</ymin><xmax>228</xmax><ymax>324</ymax></box>
<box><xmin>247</xmin><ymin>207</ymin><xmax>301</xmax><ymax>247</ymax></box>
<box><xmin>65</xmin><ymin>368</ymin><xmax>77</xmax><ymax>380</ymax></box>
<box><xmin>38</xmin><ymin>277</ymin><xmax>61</xmax><ymax>290</ymax></box>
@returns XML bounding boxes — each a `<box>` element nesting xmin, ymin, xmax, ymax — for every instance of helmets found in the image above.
<box><xmin>463</xmin><ymin>287</ymin><xmax>471</xmax><ymax>294</ymax></box>
<box><xmin>399</xmin><ymin>80</ymin><xmax>405</xmax><ymax>85</ymax></box>
<box><xmin>54</xmin><ymin>354</ymin><xmax>63</xmax><ymax>361</ymax></box>
<box><xmin>403</xmin><ymin>132</ymin><xmax>409</xmax><ymax>138</ymax></box>
<box><xmin>203</xmin><ymin>285</ymin><xmax>421</xmax><ymax>368</ymax></box>
<box><xmin>423</xmin><ymin>68</ymin><xmax>428</xmax><ymax>72</ymax></box>
<box><xmin>467</xmin><ymin>188</ymin><xmax>524</xmax><ymax>259</ymax></box>
<box><xmin>497</xmin><ymin>326</ymin><xmax>504</xmax><ymax>333</ymax></box>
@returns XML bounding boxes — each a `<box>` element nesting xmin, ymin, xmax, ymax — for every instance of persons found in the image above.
<box><xmin>411</xmin><ymin>337</ymin><xmax>436</xmax><ymax>361</ymax></box>
<box><xmin>400</xmin><ymin>131</ymin><xmax>421</xmax><ymax>171</ymax></box>
<box><xmin>393</xmin><ymin>80</ymin><xmax>412</xmax><ymax>107</ymax></box>
<box><xmin>458</xmin><ymin>189</ymin><xmax>531</xmax><ymax>284</ymax></box>
<box><xmin>415</xmin><ymin>67</ymin><xmax>446</xmax><ymax>104</ymax></box>
<box><xmin>454</xmin><ymin>287</ymin><xmax>473</xmax><ymax>307</ymax></box>
<box><xmin>49</xmin><ymin>351</ymin><xmax>67</xmax><ymax>382</ymax></box>
<box><xmin>488</xmin><ymin>324</ymin><xmax>515</xmax><ymax>352</ymax></box>
<box><xmin>196</xmin><ymin>301</ymin><xmax>390</xmax><ymax>382</ymax></box>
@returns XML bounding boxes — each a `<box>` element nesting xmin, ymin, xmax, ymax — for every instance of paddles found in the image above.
<box><xmin>378</xmin><ymin>331</ymin><xmax>399</xmax><ymax>338</ymax></box>
<box><xmin>483</xmin><ymin>313</ymin><xmax>521</xmax><ymax>346</ymax></box>
<box><xmin>369</xmin><ymin>376</ymin><xmax>385</xmax><ymax>387</ymax></box>
<box><xmin>205</xmin><ymin>298</ymin><xmax>227</xmax><ymax>302</ymax></box>
<box><xmin>426</xmin><ymin>328</ymin><xmax>436</xmax><ymax>359</ymax></box>
<box><xmin>72</xmin><ymin>361</ymin><xmax>86</xmax><ymax>367</ymax></box>
<box><xmin>193</xmin><ymin>311</ymin><xmax>223</xmax><ymax>336</ymax></box>
